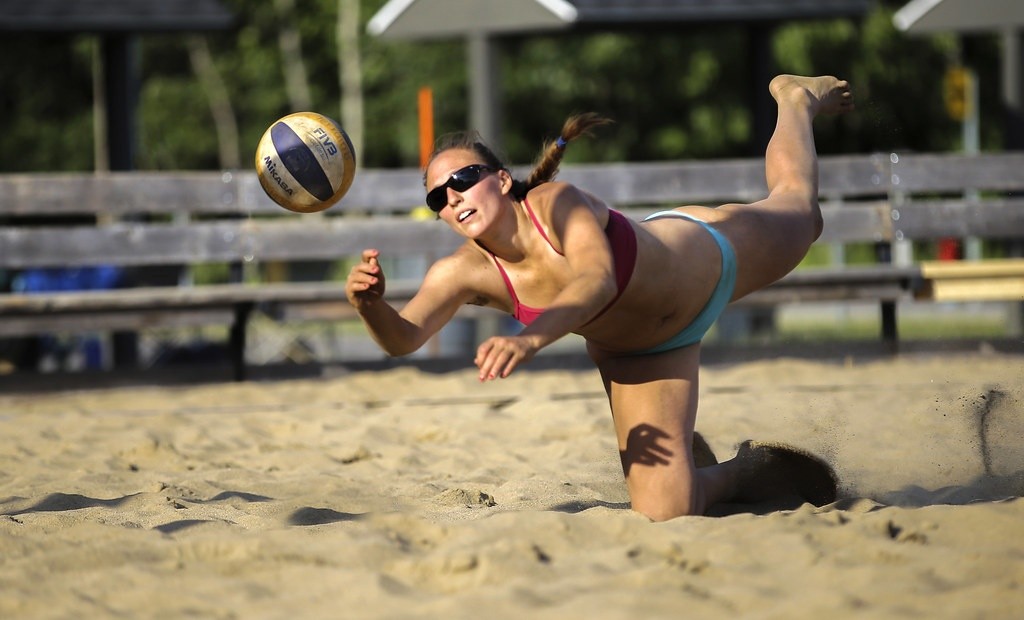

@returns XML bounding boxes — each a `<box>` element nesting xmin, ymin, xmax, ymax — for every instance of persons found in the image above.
<box><xmin>344</xmin><ymin>73</ymin><xmax>853</xmax><ymax>523</ymax></box>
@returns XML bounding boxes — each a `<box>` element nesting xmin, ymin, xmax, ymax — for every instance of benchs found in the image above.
<box><xmin>0</xmin><ymin>153</ymin><xmax>1024</xmax><ymax>387</ymax></box>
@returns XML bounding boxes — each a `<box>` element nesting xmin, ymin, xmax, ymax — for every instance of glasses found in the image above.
<box><xmin>426</xmin><ymin>164</ymin><xmax>487</xmax><ymax>212</ymax></box>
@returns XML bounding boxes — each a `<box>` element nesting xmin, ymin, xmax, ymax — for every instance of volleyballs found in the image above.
<box><xmin>255</xmin><ymin>112</ymin><xmax>359</xmax><ymax>212</ymax></box>
